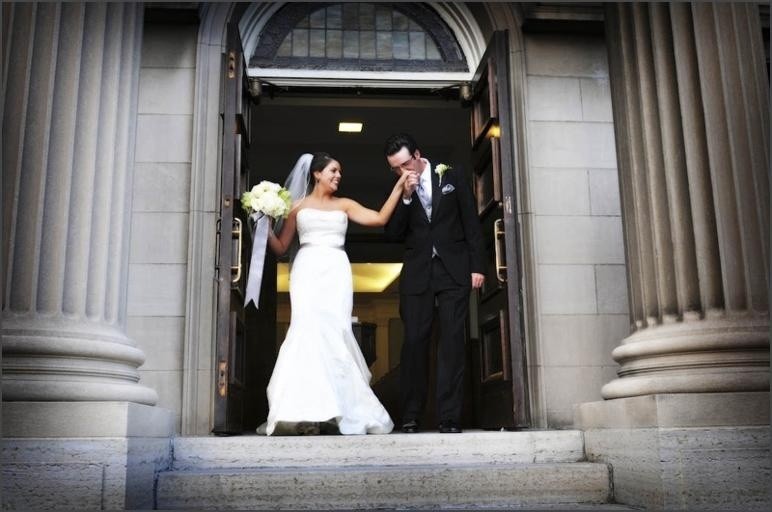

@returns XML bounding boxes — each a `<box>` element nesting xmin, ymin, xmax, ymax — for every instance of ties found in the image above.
<box><xmin>416</xmin><ymin>178</ymin><xmax>432</xmax><ymax>220</ymax></box>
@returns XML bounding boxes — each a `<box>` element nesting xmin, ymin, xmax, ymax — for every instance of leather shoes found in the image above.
<box><xmin>399</xmin><ymin>417</ymin><xmax>418</xmax><ymax>433</ymax></box>
<box><xmin>436</xmin><ymin>421</ymin><xmax>462</xmax><ymax>433</ymax></box>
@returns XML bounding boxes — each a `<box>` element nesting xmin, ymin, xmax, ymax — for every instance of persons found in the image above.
<box><xmin>247</xmin><ymin>153</ymin><xmax>416</xmax><ymax>436</ymax></box>
<box><xmin>383</xmin><ymin>134</ymin><xmax>487</xmax><ymax>433</ymax></box>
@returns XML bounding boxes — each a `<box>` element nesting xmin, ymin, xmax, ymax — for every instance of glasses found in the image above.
<box><xmin>391</xmin><ymin>156</ymin><xmax>413</xmax><ymax>172</ymax></box>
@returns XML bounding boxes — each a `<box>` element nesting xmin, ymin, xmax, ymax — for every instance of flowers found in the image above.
<box><xmin>242</xmin><ymin>180</ymin><xmax>292</xmax><ymax>220</ymax></box>
